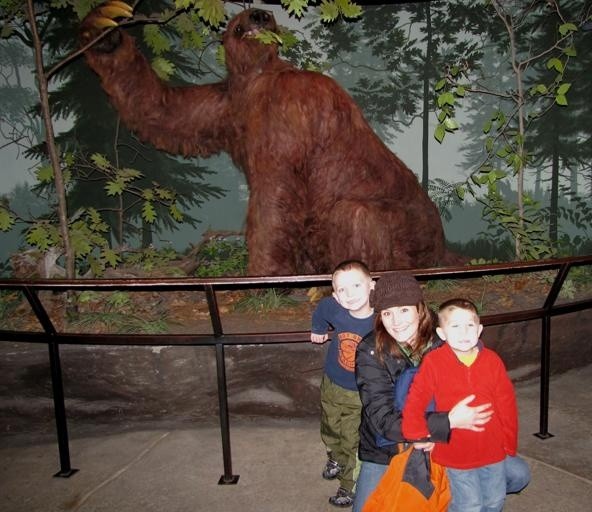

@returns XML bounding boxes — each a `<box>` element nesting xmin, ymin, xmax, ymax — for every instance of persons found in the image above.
<box><xmin>403</xmin><ymin>296</ymin><xmax>517</xmax><ymax>511</ymax></box>
<box><xmin>307</xmin><ymin>259</ymin><xmax>378</xmax><ymax>504</ymax></box>
<box><xmin>349</xmin><ymin>272</ymin><xmax>530</xmax><ymax>511</ymax></box>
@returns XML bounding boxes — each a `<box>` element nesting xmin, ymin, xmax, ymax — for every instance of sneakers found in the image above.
<box><xmin>328</xmin><ymin>486</ymin><xmax>356</xmax><ymax>507</ymax></box>
<box><xmin>322</xmin><ymin>451</ymin><xmax>343</xmax><ymax>479</ymax></box>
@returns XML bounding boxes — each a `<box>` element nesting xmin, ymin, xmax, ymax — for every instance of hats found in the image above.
<box><xmin>369</xmin><ymin>271</ymin><xmax>424</xmax><ymax>311</ymax></box>
